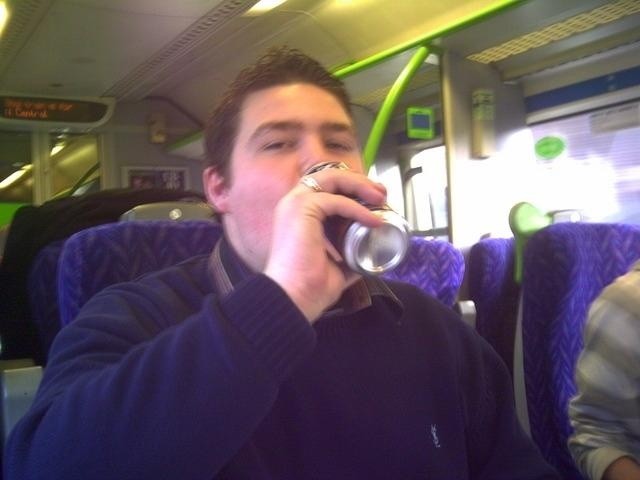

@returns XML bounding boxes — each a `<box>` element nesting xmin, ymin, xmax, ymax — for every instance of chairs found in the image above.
<box><xmin>1</xmin><ymin>189</ymin><xmax>639</xmax><ymax>479</ymax></box>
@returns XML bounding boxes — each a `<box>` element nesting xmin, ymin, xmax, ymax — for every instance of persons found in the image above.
<box><xmin>567</xmin><ymin>255</ymin><xmax>640</xmax><ymax>480</ymax></box>
<box><xmin>3</xmin><ymin>41</ymin><xmax>565</xmax><ymax>480</ymax></box>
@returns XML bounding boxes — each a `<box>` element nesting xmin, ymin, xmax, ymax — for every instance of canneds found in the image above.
<box><xmin>303</xmin><ymin>162</ymin><xmax>411</xmax><ymax>275</ymax></box>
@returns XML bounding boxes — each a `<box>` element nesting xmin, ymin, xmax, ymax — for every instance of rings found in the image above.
<box><xmin>300</xmin><ymin>175</ymin><xmax>322</xmax><ymax>192</ymax></box>
